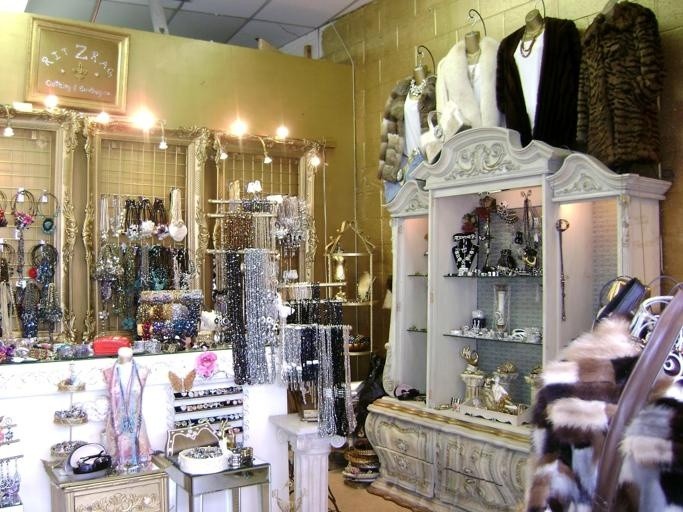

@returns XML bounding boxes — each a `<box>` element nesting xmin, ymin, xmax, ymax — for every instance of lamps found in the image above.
<box><xmin>157</xmin><ymin>119</ymin><xmax>169</xmax><ymax>150</ymax></box>
<box><xmin>307</xmin><ymin>140</ymin><xmax>321</xmax><ymax>167</ymax></box>
<box><xmin>216</xmin><ymin>136</ymin><xmax>228</xmax><ymax>160</ymax></box>
<box><xmin>257</xmin><ymin>136</ymin><xmax>273</xmax><ymax>164</ymax></box>
<box><xmin>3</xmin><ymin>106</ymin><xmax>15</xmax><ymax>138</ymax></box>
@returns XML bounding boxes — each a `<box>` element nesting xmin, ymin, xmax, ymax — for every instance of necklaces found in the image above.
<box><xmin>454</xmin><ymin>242</ymin><xmax>474</xmax><ymax>265</ymax></box>
<box><xmin>0</xmin><ymin>187</ymin><xmax>358</xmax><ymax>475</ymax></box>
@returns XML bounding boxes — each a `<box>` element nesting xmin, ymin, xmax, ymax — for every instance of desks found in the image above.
<box><xmin>268</xmin><ymin>414</ymin><xmax>332</xmax><ymax>512</ymax></box>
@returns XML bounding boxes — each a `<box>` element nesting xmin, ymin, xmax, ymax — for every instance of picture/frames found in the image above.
<box><xmin>24</xmin><ymin>15</ymin><xmax>130</xmax><ymax>114</ymax></box>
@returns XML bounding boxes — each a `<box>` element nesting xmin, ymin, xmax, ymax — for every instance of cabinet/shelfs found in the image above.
<box><xmin>364</xmin><ymin>127</ymin><xmax>673</xmax><ymax>512</ymax></box>
<box><xmin>150</xmin><ymin>452</ymin><xmax>271</xmax><ymax>512</ymax></box>
<box><xmin>40</xmin><ymin>459</ymin><xmax>170</xmax><ymax>512</ymax></box>
<box><xmin>323</xmin><ymin>218</ymin><xmax>381</xmax><ymax>357</ymax></box>
<box><xmin>169</xmin><ymin>372</ymin><xmax>244</xmax><ymax>444</ymax></box>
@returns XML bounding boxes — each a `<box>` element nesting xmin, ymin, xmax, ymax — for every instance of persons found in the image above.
<box><xmin>376</xmin><ymin>0</ymin><xmax>664</xmax><ymax>204</ymax></box>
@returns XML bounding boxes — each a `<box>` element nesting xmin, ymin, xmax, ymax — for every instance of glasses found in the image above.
<box><xmin>74</xmin><ymin>451</ymin><xmax>111</xmax><ymax>475</ymax></box>
